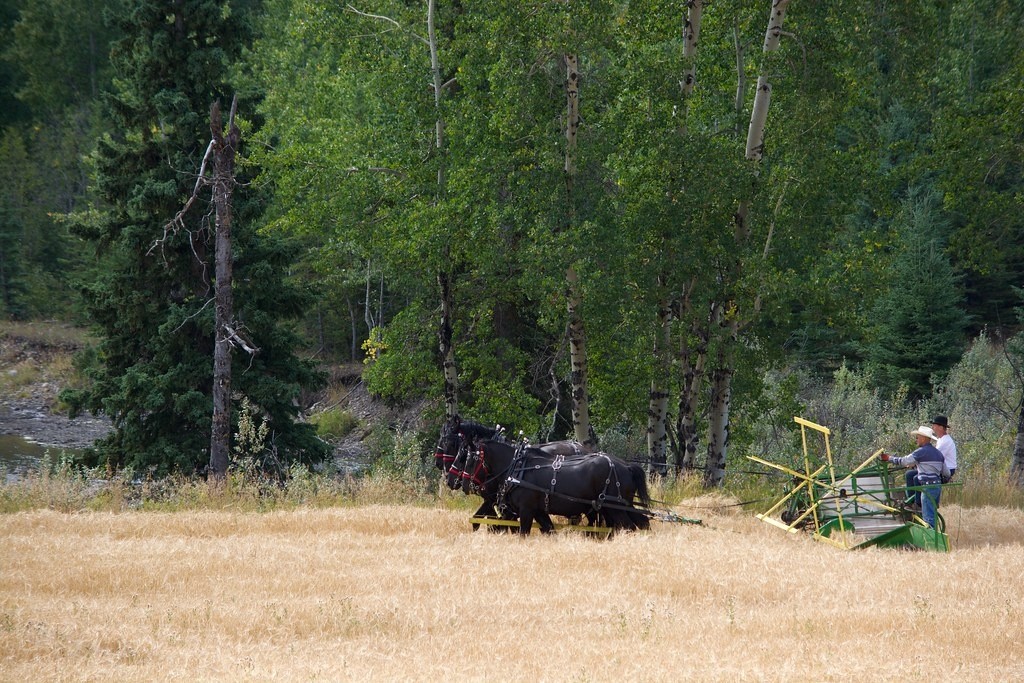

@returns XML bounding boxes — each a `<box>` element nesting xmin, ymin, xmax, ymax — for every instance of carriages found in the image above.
<box><xmin>434</xmin><ymin>411</ymin><xmax>965</xmax><ymax>552</ymax></box>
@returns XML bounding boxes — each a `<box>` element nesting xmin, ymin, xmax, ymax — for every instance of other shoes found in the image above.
<box><xmin>904</xmin><ymin>503</ymin><xmax>922</xmax><ymax>510</ymax></box>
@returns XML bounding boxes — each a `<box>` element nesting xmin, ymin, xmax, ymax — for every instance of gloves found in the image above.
<box><xmin>881</xmin><ymin>454</ymin><xmax>889</xmax><ymax>461</ymax></box>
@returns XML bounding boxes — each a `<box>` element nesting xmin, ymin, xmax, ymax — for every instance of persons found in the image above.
<box><xmin>904</xmin><ymin>416</ymin><xmax>956</xmax><ymax>512</ymax></box>
<box><xmin>881</xmin><ymin>426</ymin><xmax>950</xmax><ymax>529</ymax></box>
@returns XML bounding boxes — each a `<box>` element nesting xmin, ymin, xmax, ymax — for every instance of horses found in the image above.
<box><xmin>433</xmin><ymin>413</ymin><xmax>652</xmax><ymax>541</ymax></box>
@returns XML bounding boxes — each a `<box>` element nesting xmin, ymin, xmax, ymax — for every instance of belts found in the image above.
<box><xmin>921</xmin><ymin>480</ymin><xmax>941</xmax><ymax>484</ymax></box>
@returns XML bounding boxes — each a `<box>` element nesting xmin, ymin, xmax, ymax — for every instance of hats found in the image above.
<box><xmin>911</xmin><ymin>426</ymin><xmax>938</xmax><ymax>441</ymax></box>
<box><xmin>929</xmin><ymin>416</ymin><xmax>951</xmax><ymax>428</ymax></box>
